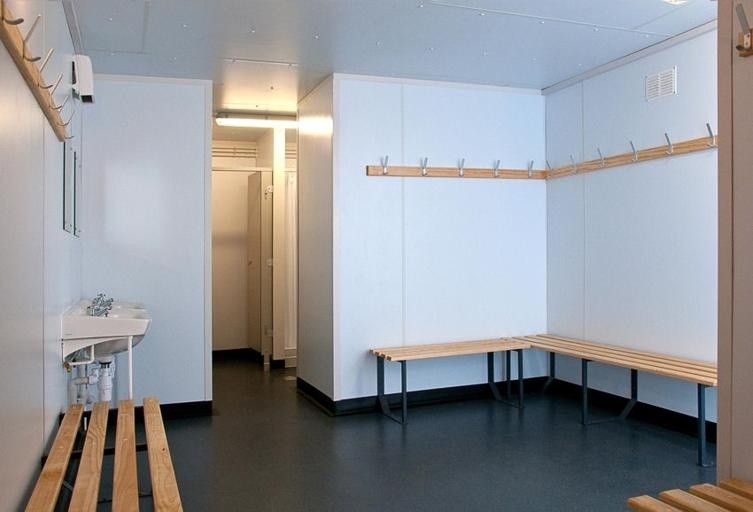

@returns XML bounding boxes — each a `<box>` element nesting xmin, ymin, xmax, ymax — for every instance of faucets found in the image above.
<box><xmin>87</xmin><ymin>293</ymin><xmax>114</xmax><ymax>316</ymax></box>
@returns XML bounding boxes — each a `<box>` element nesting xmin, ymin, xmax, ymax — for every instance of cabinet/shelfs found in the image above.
<box><xmin>718</xmin><ymin>0</ymin><xmax>753</xmax><ymax>485</ymax></box>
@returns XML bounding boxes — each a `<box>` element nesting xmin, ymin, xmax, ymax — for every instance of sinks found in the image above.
<box><xmin>112</xmin><ymin>302</ymin><xmax>143</xmax><ymax>310</ymax></box>
<box><xmin>62</xmin><ymin>310</ymin><xmax>152</xmax><ymax>366</ymax></box>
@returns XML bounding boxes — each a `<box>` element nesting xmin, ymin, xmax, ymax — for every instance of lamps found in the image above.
<box><xmin>216</xmin><ymin>112</ymin><xmax>296</xmax><ymax>129</ymax></box>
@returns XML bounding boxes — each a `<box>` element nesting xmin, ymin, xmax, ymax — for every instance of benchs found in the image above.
<box><xmin>501</xmin><ymin>335</ymin><xmax>718</xmax><ymax>467</ymax></box>
<box><xmin>627</xmin><ymin>478</ymin><xmax>753</xmax><ymax>512</ymax></box>
<box><xmin>370</xmin><ymin>337</ymin><xmax>531</xmax><ymax>424</ymax></box>
<box><xmin>24</xmin><ymin>398</ymin><xmax>183</xmax><ymax>512</ymax></box>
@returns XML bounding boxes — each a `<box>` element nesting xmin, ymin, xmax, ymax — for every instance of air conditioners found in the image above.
<box><xmin>72</xmin><ymin>54</ymin><xmax>94</xmax><ymax>104</ymax></box>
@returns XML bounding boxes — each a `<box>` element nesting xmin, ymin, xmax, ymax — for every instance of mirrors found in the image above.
<box><xmin>74</xmin><ymin>150</ymin><xmax>82</xmax><ymax>238</ymax></box>
<box><xmin>64</xmin><ymin>142</ymin><xmax>74</xmax><ymax>235</ymax></box>
